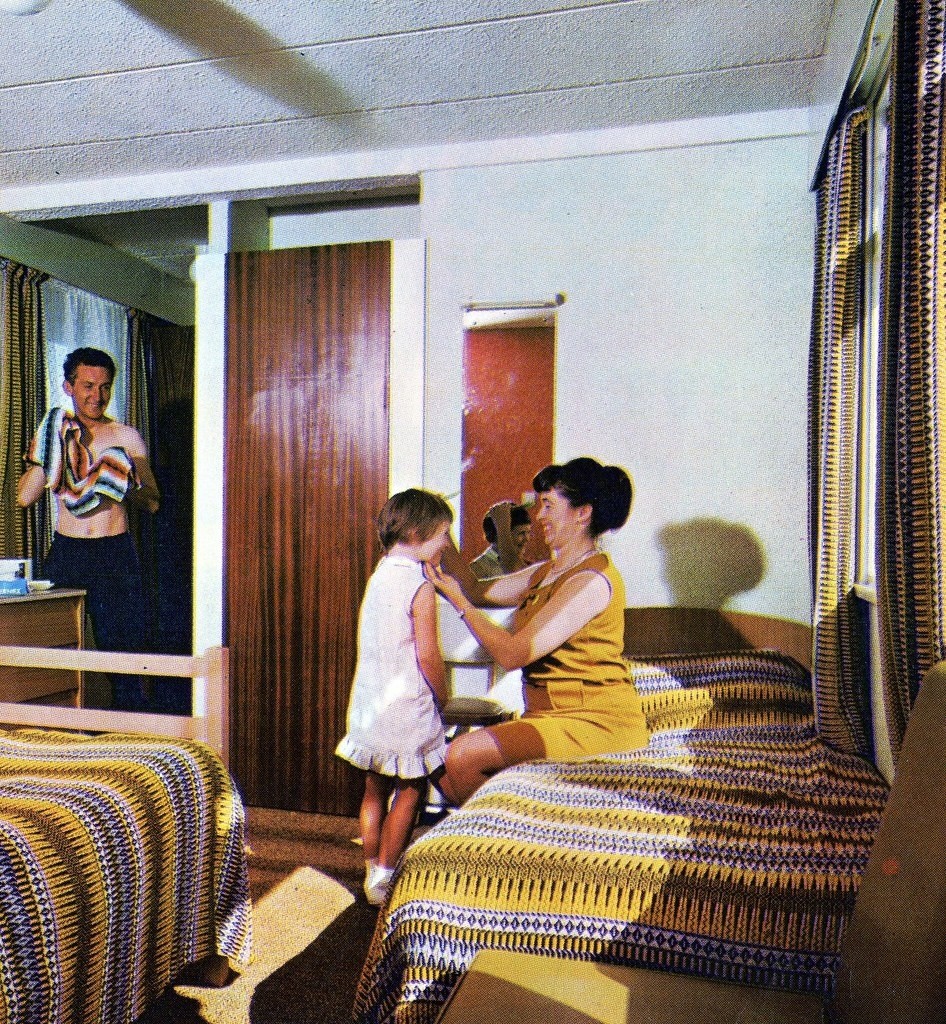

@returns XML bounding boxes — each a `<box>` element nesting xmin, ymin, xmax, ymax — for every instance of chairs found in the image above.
<box><xmin>435</xmin><ymin>658</ymin><xmax>946</xmax><ymax>1024</ymax></box>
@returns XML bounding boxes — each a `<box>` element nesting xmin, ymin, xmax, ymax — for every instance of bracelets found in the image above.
<box><xmin>456</xmin><ymin>604</ymin><xmax>474</xmax><ymax>619</ymax></box>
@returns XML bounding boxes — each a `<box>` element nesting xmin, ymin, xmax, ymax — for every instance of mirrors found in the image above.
<box><xmin>460</xmin><ymin>302</ymin><xmax>558</xmax><ymax>582</ymax></box>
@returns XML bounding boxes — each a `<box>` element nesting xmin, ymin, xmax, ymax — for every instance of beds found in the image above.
<box><xmin>351</xmin><ymin>608</ymin><xmax>895</xmax><ymax>1024</ymax></box>
<box><xmin>0</xmin><ymin>644</ymin><xmax>253</xmax><ymax>1024</ymax></box>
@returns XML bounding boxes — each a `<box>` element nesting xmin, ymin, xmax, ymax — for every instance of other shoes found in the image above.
<box><xmin>363</xmin><ymin>873</ymin><xmax>387</xmax><ymax>904</ymax></box>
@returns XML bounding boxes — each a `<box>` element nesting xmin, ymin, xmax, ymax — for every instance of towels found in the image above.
<box><xmin>22</xmin><ymin>408</ymin><xmax>143</xmax><ymax>519</ymax></box>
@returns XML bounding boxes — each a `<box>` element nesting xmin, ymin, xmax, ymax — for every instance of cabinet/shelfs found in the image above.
<box><xmin>0</xmin><ymin>587</ymin><xmax>86</xmax><ymax>736</ymax></box>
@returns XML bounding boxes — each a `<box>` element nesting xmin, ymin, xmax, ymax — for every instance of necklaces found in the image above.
<box><xmin>550</xmin><ymin>548</ymin><xmax>595</xmax><ymax>573</ymax></box>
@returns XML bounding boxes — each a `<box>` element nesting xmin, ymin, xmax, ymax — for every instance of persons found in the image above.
<box><xmin>335</xmin><ymin>488</ymin><xmax>453</xmax><ymax>905</ymax></box>
<box><xmin>17</xmin><ymin>347</ymin><xmax>161</xmax><ymax>712</ymax></box>
<box><xmin>470</xmin><ymin>501</ymin><xmax>535</xmax><ymax>579</ymax></box>
<box><xmin>423</xmin><ymin>456</ymin><xmax>649</xmax><ymax>806</ymax></box>
